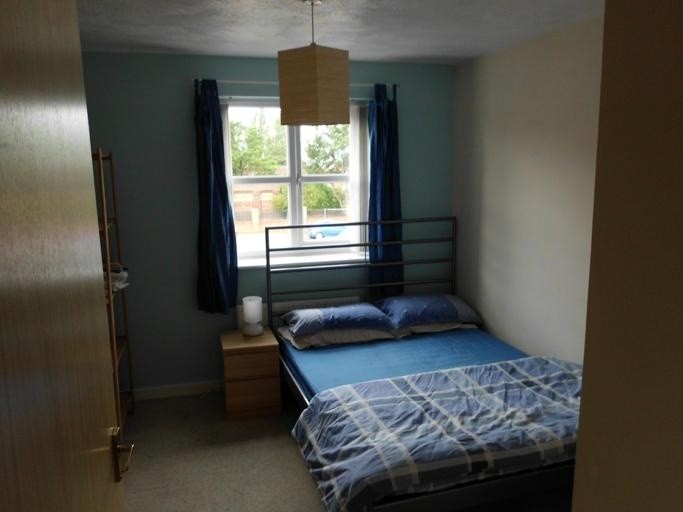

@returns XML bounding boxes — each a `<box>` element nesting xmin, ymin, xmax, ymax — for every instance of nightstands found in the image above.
<box><xmin>221</xmin><ymin>330</ymin><xmax>283</xmax><ymax>417</ymax></box>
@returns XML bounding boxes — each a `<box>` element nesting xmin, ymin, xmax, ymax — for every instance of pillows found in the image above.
<box><xmin>281</xmin><ymin>290</ymin><xmax>482</xmax><ymax>350</ymax></box>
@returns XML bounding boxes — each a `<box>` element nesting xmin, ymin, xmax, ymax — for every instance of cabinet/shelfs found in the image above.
<box><xmin>92</xmin><ymin>147</ymin><xmax>136</xmax><ymax>440</ymax></box>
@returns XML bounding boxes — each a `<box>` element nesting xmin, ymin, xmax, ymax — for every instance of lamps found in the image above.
<box><xmin>273</xmin><ymin>0</ymin><xmax>354</xmax><ymax>130</ymax></box>
<box><xmin>240</xmin><ymin>295</ymin><xmax>264</xmax><ymax>338</ymax></box>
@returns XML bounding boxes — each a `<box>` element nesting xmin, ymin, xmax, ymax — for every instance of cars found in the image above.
<box><xmin>307</xmin><ymin>219</ymin><xmax>348</xmax><ymax>239</ymax></box>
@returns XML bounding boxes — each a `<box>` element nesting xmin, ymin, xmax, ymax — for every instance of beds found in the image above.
<box><xmin>264</xmin><ymin>307</ymin><xmax>585</xmax><ymax>510</ymax></box>
